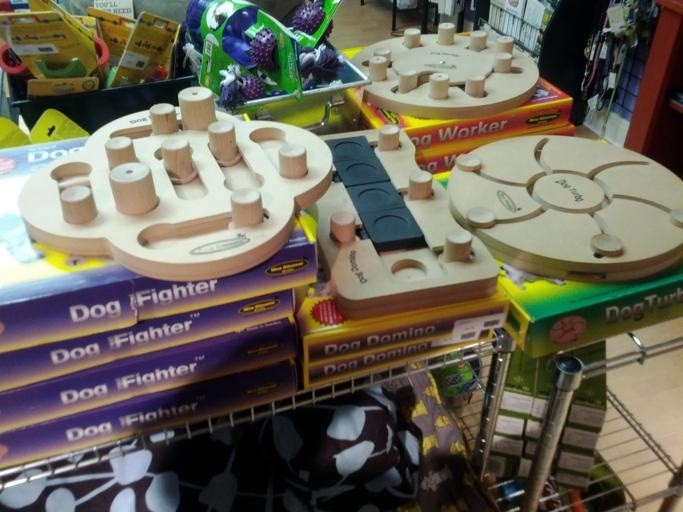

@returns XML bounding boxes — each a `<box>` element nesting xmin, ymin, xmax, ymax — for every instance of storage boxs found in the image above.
<box><xmin>231</xmin><ymin>57</ymin><xmax>372</xmax><ymax>135</ymax></box>
<box><xmin>7</xmin><ymin>64</ymin><xmax>197</xmax><ymax>136</ymax></box>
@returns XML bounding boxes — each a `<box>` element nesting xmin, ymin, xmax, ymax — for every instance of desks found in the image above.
<box><xmin>361</xmin><ymin>0</ymin><xmax>426</xmax><ymax>34</ymax></box>
